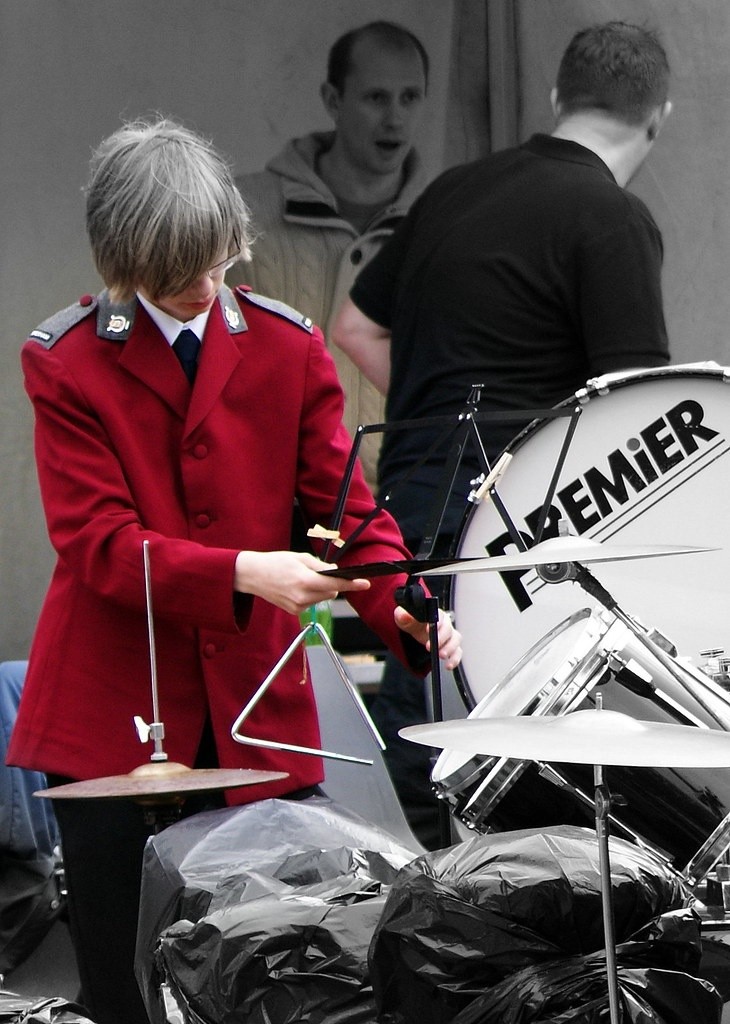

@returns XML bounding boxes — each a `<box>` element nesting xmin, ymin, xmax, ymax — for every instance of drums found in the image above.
<box><xmin>428</xmin><ymin>607</ymin><xmax>730</xmax><ymax>894</ymax></box>
<box><xmin>442</xmin><ymin>358</ymin><xmax>730</xmax><ymax>715</ymax></box>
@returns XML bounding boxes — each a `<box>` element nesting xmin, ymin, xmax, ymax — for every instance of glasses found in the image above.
<box><xmin>204</xmin><ymin>227</ymin><xmax>242</xmax><ymax>279</ymax></box>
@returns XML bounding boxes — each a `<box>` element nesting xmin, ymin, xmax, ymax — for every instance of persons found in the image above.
<box><xmin>8</xmin><ymin>114</ymin><xmax>460</xmax><ymax>1017</ymax></box>
<box><xmin>337</xmin><ymin>19</ymin><xmax>677</xmax><ymax>851</ymax></box>
<box><xmin>219</xmin><ymin>20</ymin><xmax>446</xmax><ymax>497</ymax></box>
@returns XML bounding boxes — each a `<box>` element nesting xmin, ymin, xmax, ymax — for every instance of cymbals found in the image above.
<box><xmin>397</xmin><ymin>707</ymin><xmax>730</xmax><ymax>770</ymax></box>
<box><xmin>410</xmin><ymin>535</ymin><xmax>724</xmax><ymax>576</ymax></box>
<box><xmin>32</xmin><ymin>762</ymin><xmax>289</xmax><ymax>806</ymax></box>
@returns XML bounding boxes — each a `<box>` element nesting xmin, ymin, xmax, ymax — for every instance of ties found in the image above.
<box><xmin>172</xmin><ymin>329</ymin><xmax>201</xmax><ymax>384</ymax></box>
